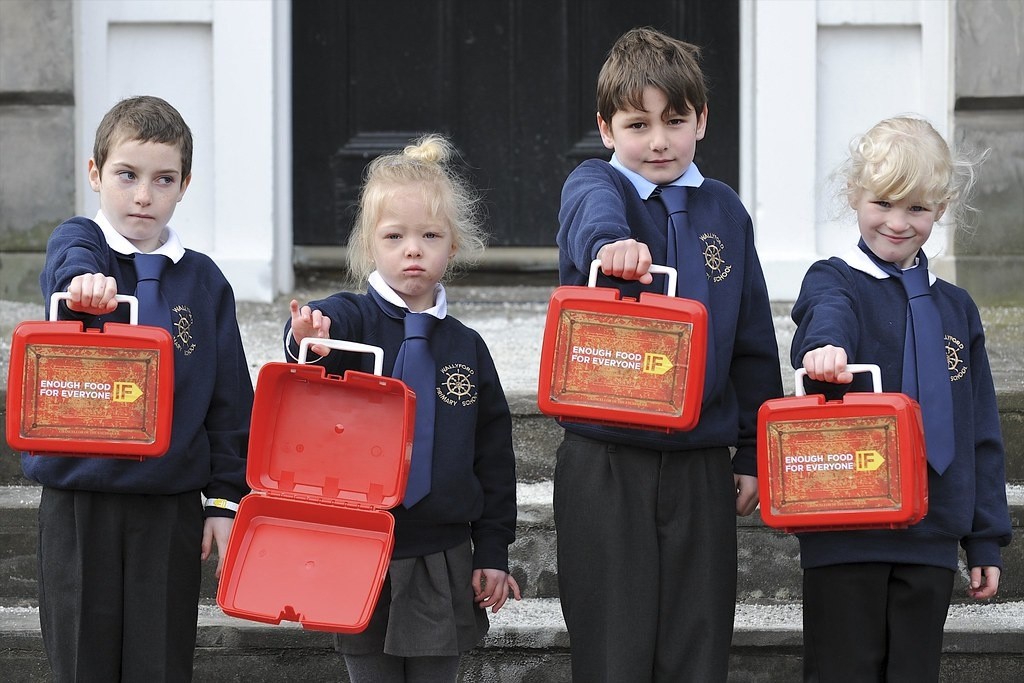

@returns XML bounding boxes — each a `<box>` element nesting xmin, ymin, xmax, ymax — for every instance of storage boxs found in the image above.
<box><xmin>756</xmin><ymin>365</ymin><xmax>927</xmax><ymax>534</ymax></box>
<box><xmin>215</xmin><ymin>337</ymin><xmax>416</xmax><ymax>633</ymax></box>
<box><xmin>5</xmin><ymin>291</ymin><xmax>173</xmax><ymax>460</ymax></box>
<box><xmin>536</xmin><ymin>260</ymin><xmax>708</xmax><ymax>438</ymax></box>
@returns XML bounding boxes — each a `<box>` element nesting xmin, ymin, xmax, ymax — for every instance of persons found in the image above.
<box><xmin>789</xmin><ymin>120</ymin><xmax>1009</xmax><ymax>683</ymax></box>
<box><xmin>550</xmin><ymin>24</ymin><xmax>784</xmax><ymax>682</ymax></box>
<box><xmin>284</xmin><ymin>133</ymin><xmax>521</xmax><ymax>682</ymax></box>
<box><xmin>20</xmin><ymin>95</ymin><xmax>255</xmax><ymax>683</ymax></box>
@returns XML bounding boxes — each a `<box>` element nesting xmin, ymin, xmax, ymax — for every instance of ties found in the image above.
<box><xmin>131</xmin><ymin>251</ymin><xmax>173</xmax><ymax>340</ymax></box>
<box><xmin>655</xmin><ymin>184</ymin><xmax>718</xmax><ymax>406</ymax></box>
<box><xmin>858</xmin><ymin>231</ymin><xmax>958</xmax><ymax>477</ymax></box>
<box><xmin>366</xmin><ymin>287</ymin><xmax>437</xmax><ymax>510</ymax></box>
